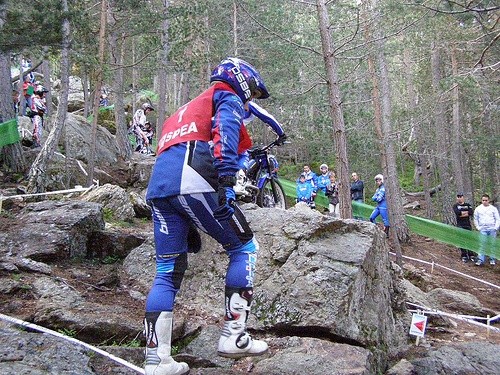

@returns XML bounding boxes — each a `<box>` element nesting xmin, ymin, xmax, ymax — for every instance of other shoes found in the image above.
<box><xmin>461</xmin><ymin>257</ymin><xmax>467</xmax><ymax>263</ymax></box>
<box><xmin>490</xmin><ymin>260</ymin><xmax>495</xmax><ymax>265</ymax></box>
<box><xmin>475</xmin><ymin>260</ymin><xmax>483</xmax><ymax>265</ymax></box>
<box><xmin>470</xmin><ymin>258</ymin><xmax>474</xmax><ymax>262</ymax></box>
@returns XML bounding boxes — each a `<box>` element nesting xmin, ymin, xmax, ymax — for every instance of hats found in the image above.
<box><xmin>457</xmin><ymin>193</ymin><xmax>463</xmax><ymax>197</ymax></box>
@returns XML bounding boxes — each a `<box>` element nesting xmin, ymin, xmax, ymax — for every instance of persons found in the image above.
<box><xmin>123</xmin><ymin>102</ymin><xmax>157</xmax><ymax>157</ymax></box>
<box><xmin>369</xmin><ymin>174</ymin><xmax>391</xmax><ymax>238</ymax></box>
<box><xmin>92</xmin><ymin>93</ymin><xmax>107</xmax><ymax>115</ymax></box>
<box><xmin>295</xmin><ymin>164</ymin><xmax>318</xmax><ymax>209</ymax></box>
<box><xmin>452</xmin><ymin>193</ymin><xmax>476</xmax><ymax>263</ymax></box>
<box><xmin>9</xmin><ymin>56</ymin><xmax>48</xmax><ymax>145</ymax></box>
<box><xmin>349</xmin><ymin>172</ymin><xmax>364</xmax><ymax>221</ymax></box>
<box><xmin>144</xmin><ymin>57</ymin><xmax>288</xmax><ymax>375</ymax></box>
<box><xmin>318</xmin><ymin>163</ymin><xmax>342</xmax><ymax>219</ymax></box>
<box><xmin>473</xmin><ymin>195</ymin><xmax>499</xmax><ymax>266</ymax></box>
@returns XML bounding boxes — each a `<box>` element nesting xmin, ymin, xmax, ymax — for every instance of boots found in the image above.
<box><xmin>142</xmin><ymin>311</ymin><xmax>190</xmax><ymax>375</ymax></box>
<box><xmin>217</xmin><ymin>287</ymin><xmax>268</xmax><ymax>358</ymax></box>
<box><xmin>33</xmin><ymin>140</ymin><xmax>40</xmax><ymax>147</ymax></box>
<box><xmin>135</xmin><ymin>140</ymin><xmax>141</xmax><ymax>151</ymax></box>
<box><xmin>233</xmin><ymin>168</ymin><xmax>252</xmax><ymax>197</ymax></box>
<box><xmin>385</xmin><ymin>227</ymin><xmax>389</xmax><ymax>238</ymax></box>
<box><xmin>147</xmin><ymin>146</ymin><xmax>155</xmax><ymax>155</ymax></box>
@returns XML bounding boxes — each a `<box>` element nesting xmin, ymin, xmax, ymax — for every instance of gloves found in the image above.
<box><xmin>213</xmin><ymin>175</ymin><xmax>237</xmax><ymax>224</ymax></box>
<box><xmin>277</xmin><ymin>134</ymin><xmax>286</xmax><ymax>146</ymax></box>
<box><xmin>187</xmin><ymin>224</ymin><xmax>201</xmax><ymax>254</ymax></box>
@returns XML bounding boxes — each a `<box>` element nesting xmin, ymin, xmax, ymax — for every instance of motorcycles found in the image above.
<box><xmin>234</xmin><ymin>135</ymin><xmax>292</xmax><ymax>210</ymax></box>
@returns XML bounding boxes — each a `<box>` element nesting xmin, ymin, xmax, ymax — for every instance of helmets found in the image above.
<box><xmin>374</xmin><ymin>174</ymin><xmax>384</xmax><ymax>184</ymax></box>
<box><xmin>210</xmin><ymin>58</ymin><xmax>269</xmax><ymax>104</ymax></box>
<box><xmin>34</xmin><ymin>84</ymin><xmax>49</xmax><ymax>92</ymax></box>
<box><xmin>142</xmin><ymin>103</ymin><xmax>154</xmax><ymax>111</ymax></box>
<box><xmin>320</xmin><ymin>164</ymin><xmax>328</xmax><ymax>172</ymax></box>
